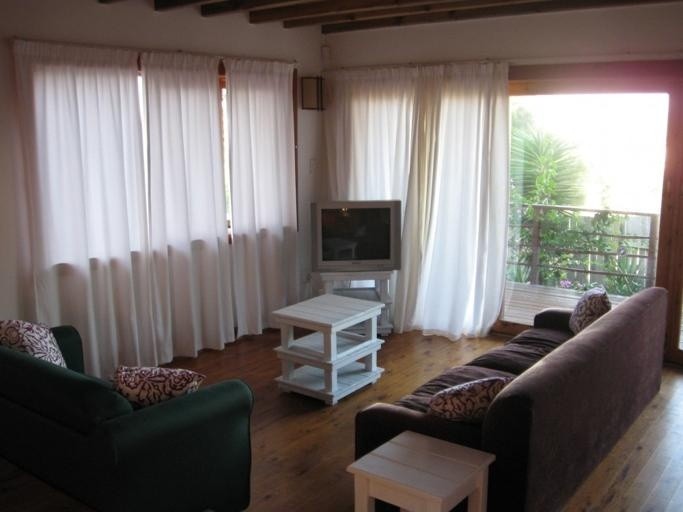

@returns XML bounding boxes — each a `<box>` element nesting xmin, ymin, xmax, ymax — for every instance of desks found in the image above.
<box><xmin>271</xmin><ymin>271</ymin><xmax>394</xmax><ymax>405</ymax></box>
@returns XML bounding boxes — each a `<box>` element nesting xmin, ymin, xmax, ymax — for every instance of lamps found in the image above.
<box><xmin>301</xmin><ymin>76</ymin><xmax>324</xmax><ymax>110</ymax></box>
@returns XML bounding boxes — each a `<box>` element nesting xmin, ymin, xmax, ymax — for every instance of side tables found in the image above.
<box><xmin>345</xmin><ymin>430</ymin><xmax>497</xmax><ymax>511</ymax></box>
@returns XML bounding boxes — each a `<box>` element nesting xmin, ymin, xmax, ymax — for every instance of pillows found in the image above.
<box><xmin>427</xmin><ymin>375</ymin><xmax>516</xmax><ymax>423</ymax></box>
<box><xmin>110</xmin><ymin>365</ymin><xmax>207</xmax><ymax>409</ymax></box>
<box><xmin>0</xmin><ymin>318</ymin><xmax>66</xmax><ymax>368</ymax></box>
<box><xmin>568</xmin><ymin>285</ymin><xmax>611</xmax><ymax>335</ymax></box>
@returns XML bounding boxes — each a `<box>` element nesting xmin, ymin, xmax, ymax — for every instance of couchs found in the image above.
<box><xmin>354</xmin><ymin>285</ymin><xmax>668</xmax><ymax>511</ymax></box>
<box><xmin>0</xmin><ymin>324</ymin><xmax>253</xmax><ymax>512</ymax></box>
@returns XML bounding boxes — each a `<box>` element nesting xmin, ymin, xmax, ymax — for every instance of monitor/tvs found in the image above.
<box><xmin>311</xmin><ymin>200</ymin><xmax>401</xmax><ymax>272</ymax></box>
